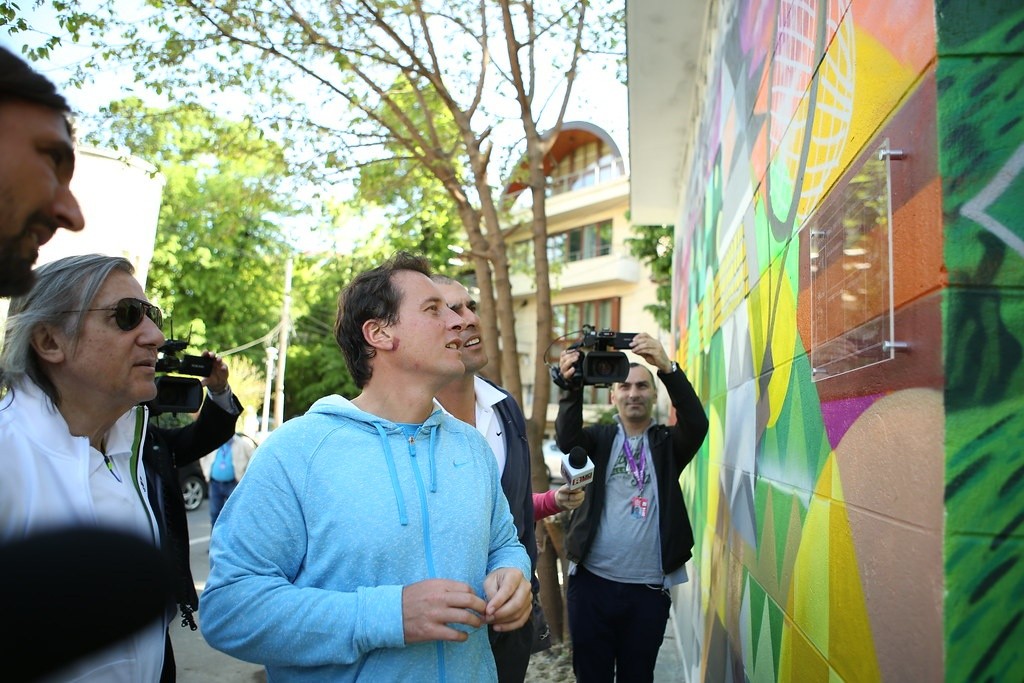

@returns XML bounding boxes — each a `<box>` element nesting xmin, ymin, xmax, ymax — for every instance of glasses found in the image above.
<box><xmin>54</xmin><ymin>298</ymin><xmax>162</xmax><ymax>331</ymax></box>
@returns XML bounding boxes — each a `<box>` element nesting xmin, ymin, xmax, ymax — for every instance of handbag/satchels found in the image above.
<box><xmin>529</xmin><ymin>601</ymin><xmax>551</xmax><ymax>655</ymax></box>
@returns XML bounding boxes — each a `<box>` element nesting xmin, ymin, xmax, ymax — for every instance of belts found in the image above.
<box><xmin>211</xmin><ymin>477</ymin><xmax>235</xmax><ymax>484</ymax></box>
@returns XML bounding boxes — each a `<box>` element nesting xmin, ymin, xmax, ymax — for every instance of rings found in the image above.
<box><xmin>568</xmin><ymin>494</ymin><xmax>570</xmax><ymax>501</ymax></box>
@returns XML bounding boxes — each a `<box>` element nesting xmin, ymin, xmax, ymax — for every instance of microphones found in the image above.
<box><xmin>560</xmin><ymin>446</ymin><xmax>597</xmax><ymax>491</ymax></box>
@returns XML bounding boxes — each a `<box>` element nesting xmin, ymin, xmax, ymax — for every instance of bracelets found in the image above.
<box><xmin>671</xmin><ymin>360</ymin><xmax>678</xmax><ymax>372</ymax></box>
<box><xmin>210</xmin><ymin>384</ymin><xmax>229</xmax><ymax>395</ymax></box>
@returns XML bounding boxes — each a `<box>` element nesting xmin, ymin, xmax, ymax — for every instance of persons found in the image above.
<box><xmin>0</xmin><ymin>43</ymin><xmax>84</xmax><ymax>300</ymax></box>
<box><xmin>633</xmin><ymin>507</ymin><xmax>642</xmax><ymax>519</ymax></box>
<box><xmin>200</xmin><ymin>249</ymin><xmax>534</xmax><ymax>683</ymax></box>
<box><xmin>141</xmin><ymin>349</ymin><xmax>245</xmax><ymax>683</ymax></box>
<box><xmin>532</xmin><ymin>481</ymin><xmax>585</xmax><ymax>520</ymax></box>
<box><xmin>201</xmin><ymin>433</ymin><xmax>255</xmax><ymax>554</ymax></box>
<box><xmin>0</xmin><ymin>252</ymin><xmax>173</xmax><ymax>683</ymax></box>
<box><xmin>432</xmin><ymin>273</ymin><xmax>537</xmax><ymax>683</ymax></box>
<box><xmin>554</xmin><ymin>332</ymin><xmax>710</xmax><ymax>683</ymax></box>
